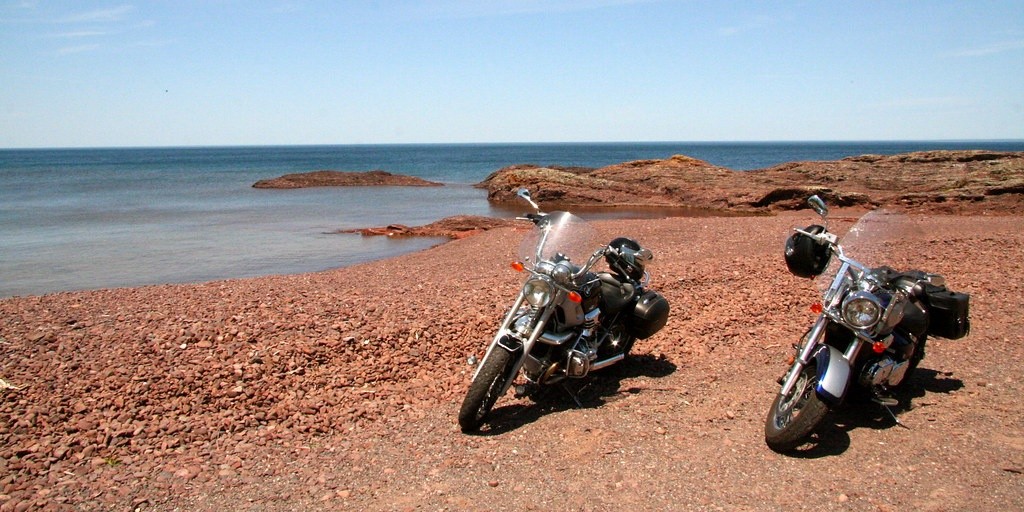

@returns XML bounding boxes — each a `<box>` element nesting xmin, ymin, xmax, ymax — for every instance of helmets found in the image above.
<box><xmin>784</xmin><ymin>224</ymin><xmax>832</xmax><ymax>279</ymax></box>
<box><xmin>604</xmin><ymin>236</ymin><xmax>646</xmax><ymax>285</ymax></box>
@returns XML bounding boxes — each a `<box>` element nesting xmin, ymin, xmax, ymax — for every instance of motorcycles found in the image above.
<box><xmin>456</xmin><ymin>186</ymin><xmax>653</xmax><ymax>429</ymax></box>
<box><xmin>762</xmin><ymin>195</ymin><xmax>948</xmax><ymax>454</ymax></box>
